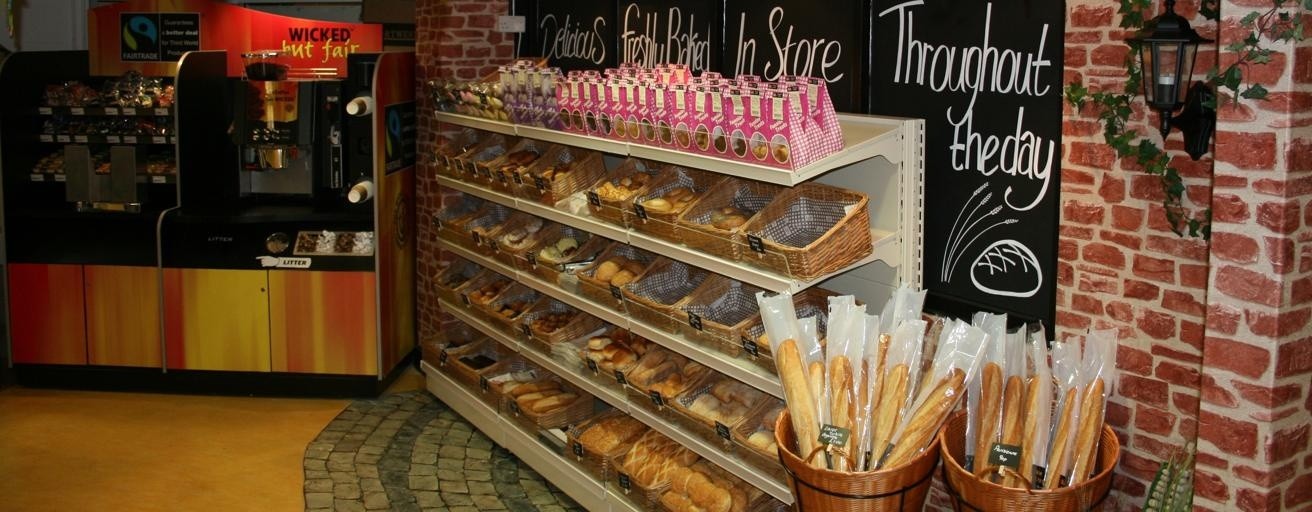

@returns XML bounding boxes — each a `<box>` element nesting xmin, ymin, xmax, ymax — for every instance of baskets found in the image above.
<box><xmin>569</xmin><ymin>152</ymin><xmax>872</xmax><ymax>512</ymax></box>
<box><xmin>436</xmin><ymin>133</ymin><xmax>568</xmax><ymax>429</ymax></box>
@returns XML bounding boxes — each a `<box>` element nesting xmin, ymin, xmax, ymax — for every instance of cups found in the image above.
<box><xmin>348</xmin><ymin>180</ymin><xmax>374</xmax><ymax>205</ymax></box>
<box><xmin>346</xmin><ymin>95</ymin><xmax>373</xmax><ymax>118</ymax></box>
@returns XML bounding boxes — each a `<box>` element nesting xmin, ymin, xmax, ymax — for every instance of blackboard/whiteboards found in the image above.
<box><xmin>508</xmin><ymin>0</ymin><xmax>1066</xmax><ymax>346</ymax></box>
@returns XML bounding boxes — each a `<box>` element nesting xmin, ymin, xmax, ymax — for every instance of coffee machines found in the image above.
<box><xmin>226</xmin><ymin>49</ymin><xmax>312</xmax><ymax>172</ymax></box>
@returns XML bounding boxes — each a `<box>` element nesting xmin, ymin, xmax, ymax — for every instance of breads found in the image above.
<box><xmin>437</xmin><ymin>149</ymin><xmax>1106</xmax><ymax>512</ymax></box>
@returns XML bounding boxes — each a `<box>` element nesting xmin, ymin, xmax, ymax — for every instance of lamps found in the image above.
<box><xmin>1120</xmin><ymin>1</ymin><xmax>1216</xmax><ymax>161</ymax></box>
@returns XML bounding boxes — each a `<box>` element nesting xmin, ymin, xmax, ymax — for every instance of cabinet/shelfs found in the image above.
<box><xmin>6</xmin><ymin>262</ymin><xmax>165</xmax><ymax>394</ymax></box>
<box><xmin>1</xmin><ymin>49</ymin><xmax>228</xmax><ymax>266</ymax></box>
<box><xmin>420</xmin><ymin>61</ymin><xmax>927</xmax><ymax>512</ymax></box>
<box><xmin>161</xmin><ymin>265</ymin><xmax>414</xmax><ymax>400</ymax></box>
